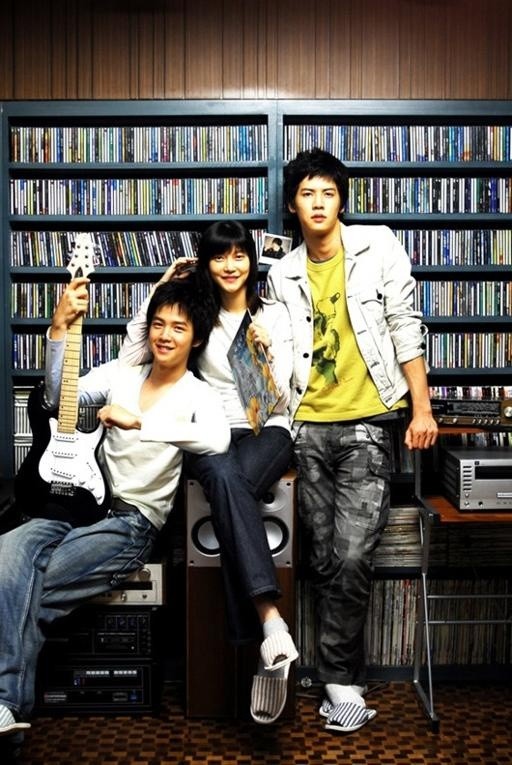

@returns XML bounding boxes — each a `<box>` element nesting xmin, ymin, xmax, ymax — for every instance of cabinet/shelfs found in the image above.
<box><xmin>271</xmin><ymin>94</ymin><xmax>511</xmax><ymax>689</ymax></box>
<box><xmin>404</xmin><ymin>408</ymin><xmax>510</xmax><ymax>730</ymax></box>
<box><xmin>2</xmin><ymin>93</ymin><xmax>277</xmax><ymax>495</ymax></box>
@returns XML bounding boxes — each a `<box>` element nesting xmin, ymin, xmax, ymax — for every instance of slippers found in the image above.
<box><xmin>318</xmin><ymin>700</ymin><xmax>333</xmax><ymax>718</ymax></box>
<box><xmin>1</xmin><ymin>705</ymin><xmax>32</xmax><ymax>738</ymax></box>
<box><xmin>250</xmin><ymin>659</ymin><xmax>292</xmax><ymax>725</ymax></box>
<box><xmin>259</xmin><ymin>630</ymin><xmax>300</xmax><ymax>672</ymax></box>
<box><xmin>324</xmin><ymin>703</ymin><xmax>377</xmax><ymax>732</ymax></box>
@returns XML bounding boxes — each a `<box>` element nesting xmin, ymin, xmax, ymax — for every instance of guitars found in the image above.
<box><xmin>11</xmin><ymin>234</ymin><xmax>110</xmax><ymax>527</ymax></box>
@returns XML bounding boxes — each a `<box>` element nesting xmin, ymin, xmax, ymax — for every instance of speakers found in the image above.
<box><xmin>184</xmin><ymin>467</ymin><xmax>298</xmax><ymax>719</ymax></box>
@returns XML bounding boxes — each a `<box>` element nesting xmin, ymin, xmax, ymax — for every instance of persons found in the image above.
<box><xmin>117</xmin><ymin>221</ymin><xmax>300</xmax><ymax>727</ymax></box>
<box><xmin>0</xmin><ymin>276</ymin><xmax>233</xmax><ymax>733</ymax></box>
<box><xmin>262</xmin><ymin>238</ymin><xmax>286</xmax><ymax>260</ymax></box>
<box><xmin>266</xmin><ymin>148</ymin><xmax>439</xmax><ymax>711</ymax></box>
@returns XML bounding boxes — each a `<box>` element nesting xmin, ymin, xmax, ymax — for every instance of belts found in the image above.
<box><xmin>109</xmin><ymin>497</ymin><xmax>138</xmax><ymax>512</ymax></box>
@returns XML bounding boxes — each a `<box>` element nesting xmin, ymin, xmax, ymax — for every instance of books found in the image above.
<box><xmin>294</xmin><ymin>507</ymin><xmax>512</xmax><ymax>670</ymax></box>
<box><xmin>226</xmin><ymin>308</ymin><xmax>284</xmax><ymax>437</ymax></box>
<box><xmin>12</xmin><ymin>385</ymin><xmax>107</xmax><ymax>477</ymax></box>
